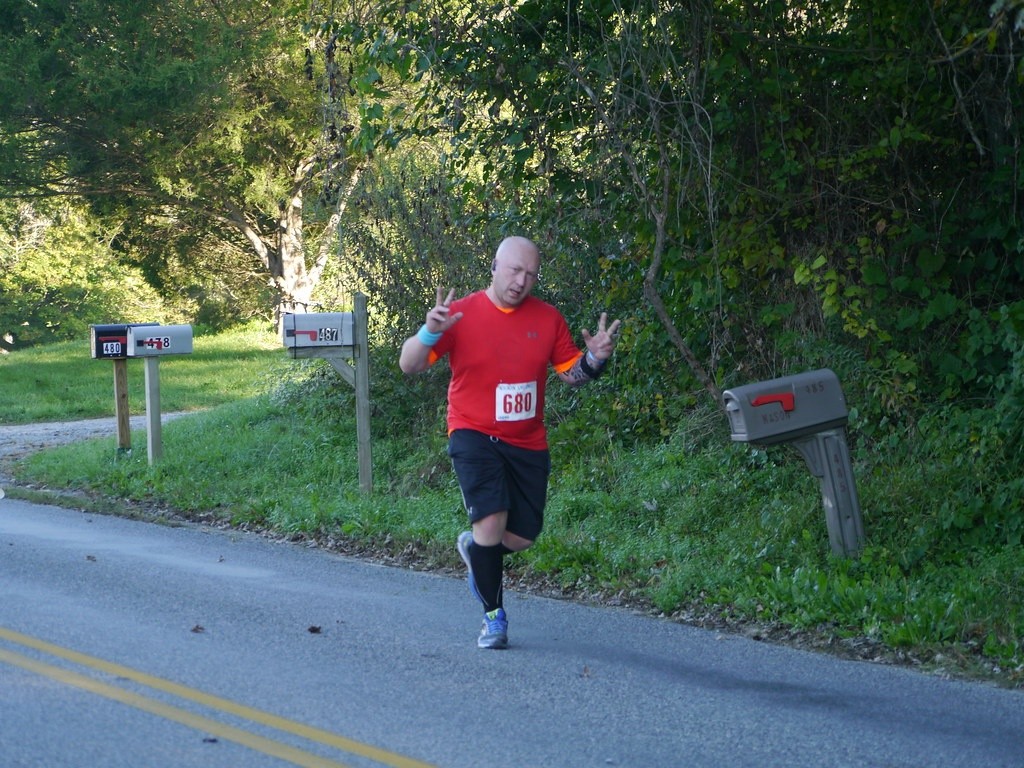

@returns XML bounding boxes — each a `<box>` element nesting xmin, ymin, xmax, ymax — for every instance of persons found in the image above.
<box><xmin>398</xmin><ymin>235</ymin><xmax>621</xmax><ymax>649</ymax></box>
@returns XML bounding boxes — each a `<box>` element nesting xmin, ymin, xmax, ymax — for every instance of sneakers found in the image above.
<box><xmin>458</xmin><ymin>531</ymin><xmax>474</xmax><ymax>595</ymax></box>
<box><xmin>478</xmin><ymin>608</ymin><xmax>509</xmax><ymax>648</ymax></box>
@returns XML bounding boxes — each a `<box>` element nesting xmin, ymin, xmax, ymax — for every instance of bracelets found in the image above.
<box><xmin>418</xmin><ymin>324</ymin><xmax>442</xmax><ymax>347</ymax></box>
<box><xmin>580</xmin><ymin>350</ymin><xmax>601</xmax><ymax>380</ymax></box>
<box><xmin>588</xmin><ymin>350</ymin><xmax>606</xmax><ymax>365</ymax></box>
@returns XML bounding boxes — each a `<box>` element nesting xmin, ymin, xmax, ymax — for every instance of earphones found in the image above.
<box><xmin>492</xmin><ymin>263</ymin><xmax>496</xmax><ymax>271</ymax></box>
